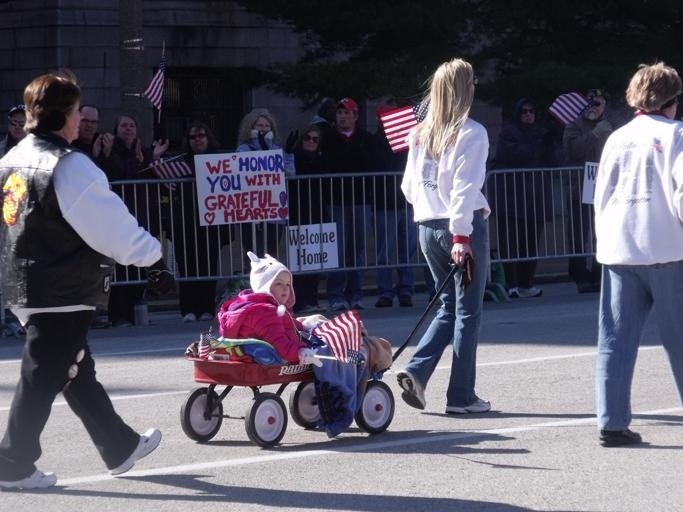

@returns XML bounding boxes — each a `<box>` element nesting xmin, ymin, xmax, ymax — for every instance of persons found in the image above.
<box><xmin>107</xmin><ymin>115</ymin><xmax>150</xmax><ymax>328</ymax></box>
<box><xmin>328</xmin><ymin>97</ymin><xmax>368</xmax><ymax>311</ymax></box>
<box><xmin>563</xmin><ymin>83</ymin><xmax>620</xmax><ymax>296</ymax></box>
<box><xmin>487</xmin><ymin>98</ymin><xmax>560</xmax><ymax>297</ymax></box>
<box><xmin>394</xmin><ymin>59</ymin><xmax>491</xmax><ymax>418</ymax></box>
<box><xmin>174</xmin><ymin>120</ymin><xmax>233</xmax><ymax>321</ymax></box>
<box><xmin>232</xmin><ymin>109</ymin><xmax>296</xmax><ymax>271</ymax></box>
<box><xmin>592</xmin><ymin>62</ymin><xmax>683</xmax><ymax>448</ymax></box>
<box><xmin>0</xmin><ymin>73</ymin><xmax>163</xmax><ymax>489</ymax></box>
<box><xmin>220</xmin><ymin>251</ymin><xmax>317</xmax><ymax>362</ymax></box>
<box><xmin>3</xmin><ymin>105</ymin><xmax>32</xmax><ymax>160</ymax></box>
<box><xmin>78</xmin><ymin>102</ymin><xmax>124</xmax><ymax>186</ymax></box>
<box><xmin>373</xmin><ymin>118</ymin><xmax>422</xmax><ymax>308</ymax></box>
<box><xmin>292</xmin><ymin>125</ymin><xmax>329</xmax><ymax>314</ymax></box>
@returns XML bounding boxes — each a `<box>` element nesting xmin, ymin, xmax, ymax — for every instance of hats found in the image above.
<box><xmin>9</xmin><ymin>104</ymin><xmax>27</xmax><ymax>115</ymax></box>
<box><xmin>247</xmin><ymin>251</ymin><xmax>296</xmax><ymax>313</ymax></box>
<box><xmin>338</xmin><ymin>97</ymin><xmax>359</xmax><ymax>110</ymax></box>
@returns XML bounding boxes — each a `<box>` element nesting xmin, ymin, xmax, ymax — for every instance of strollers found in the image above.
<box><xmin>178</xmin><ymin>252</ymin><xmax>474</xmax><ymax>449</ymax></box>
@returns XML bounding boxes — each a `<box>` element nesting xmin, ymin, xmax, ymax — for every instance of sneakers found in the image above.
<box><xmin>0</xmin><ymin>469</ymin><xmax>58</xmax><ymax>489</ymax></box>
<box><xmin>318</xmin><ymin>304</ymin><xmax>326</xmax><ymax>312</ymax></box>
<box><xmin>508</xmin><ymin>287</ymin><xmax>520</xmax><ymax>297</ymax></box>
<box><xmin>299</xmin><ymin>305</ymin><xmax>317</xmax><ymax>313</ymax></box>
<box><xmin>521</xmin><ymin>288</ymin><xmax>542</xmax><ymax>297</ymax></box>
<box><xmin>351</xmin><ymin>300</ymin><xmax>365</xmax><ymax>310</ymax></box>
<box><xmin>183</xmin><ymin>313</ymin><xmax>196</xmax><ymax>321</ymax></box>
<box><xmin>328</xmin><ymin>301</ymin><xmax>348</xmax><ymax>310</ymax></box>
<box><xmin>599</xmin><ymin>428</ymin><xmax>642</xmax><ymax>447</ymax></box>
<box><xmin>200</xmin><ymin>313</ymin><xmax>213</xmax><ymax>321</ymax></box>
<box><xmin>446</xmin><ymin>392</ymin><xmax>491</xmax><ymax>414</ymax></box>
<box><xmin>108</xmin><ymin>429</ymin><xmax>162</xmax><ymax>474</ymax></box>
<box><xmin>397</xmin><ymin>370</ymin><xmax>426</xmax><ymax>409</ymax></box>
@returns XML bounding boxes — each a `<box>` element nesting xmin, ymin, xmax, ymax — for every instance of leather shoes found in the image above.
<box><xmin>375</xmin><ymin>296</ymin><xmax>392</xmax><ymax>307</ymax></box>
<box><xmin>399</xmin><ymin>295</ymin><xmax>413</xmax><ymax>307</ymax></box>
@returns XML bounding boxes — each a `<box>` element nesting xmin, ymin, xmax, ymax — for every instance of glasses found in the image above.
<box><xmin>303</xmin><ymin>134</ymin><xmax>320</xmax><ymax>144</ymax></box>
<box><xmin>81</xmin><ymin>118</ymin><xmax>97</xmax><ymax>126</ymax></box>
<box><xmin>522</xmin><ymin>107</ymin><xmax>535</xmax><ymax>115</ymax></box>
<box><xmin>190</xmin><ymin>133</ymin><xmax>207</xmax><ymax>140</ymax></box>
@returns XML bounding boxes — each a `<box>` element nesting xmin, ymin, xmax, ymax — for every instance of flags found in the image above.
<box><xmin>383</xmin><ymin>101</ymin><xmax>430</xmax><ymax>152</ymax></box>
<box><xmin>152</xmin><ymin>154</ymin><xmax>192</xmax><ymax>189</ymax></box>
<box><xmin>143</xmin><ymin>51</ymin><xmax>168</xmax><ymax>110</ymax></box>
<box><xmin>549</xmin><ymin>91</ymin><xmax>591</xmax><ymax>127</ymax></box>
